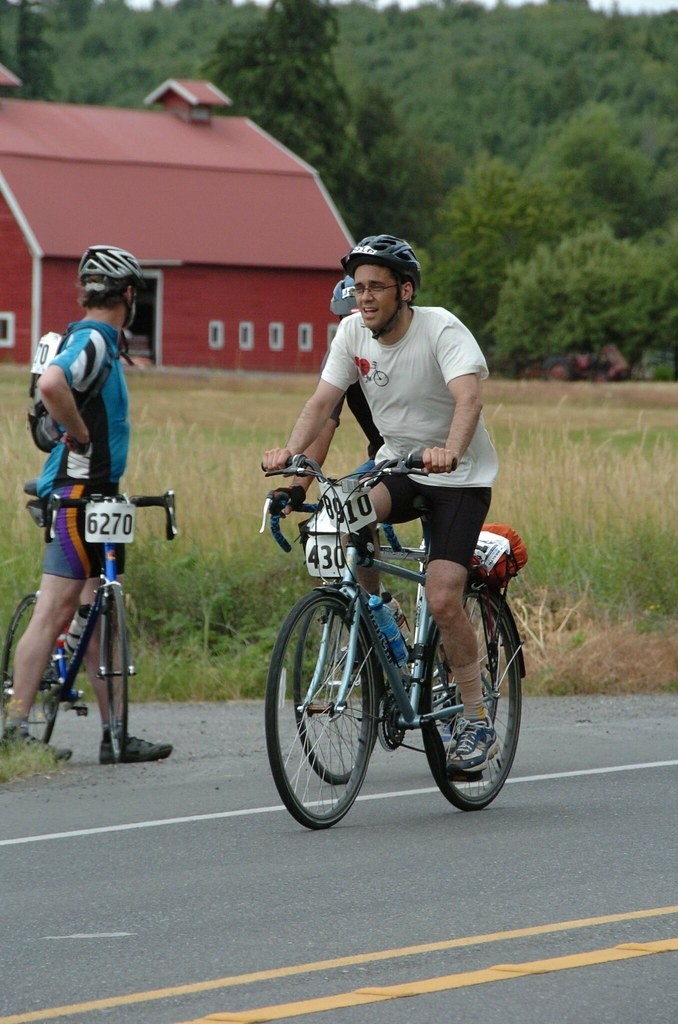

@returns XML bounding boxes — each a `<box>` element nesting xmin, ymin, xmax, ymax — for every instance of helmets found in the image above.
<box><xmin>77</xmin><ymin>243</ymin><xmax>147</xmax><ymax>290</ymax></box>
<box><xmin>329</xmin><ymin>275</ymin><xmax>362</xmax><ymax>316</ymax></box>
<box><xmin>340</xmin><ymin>234</ymin><xmax>422</xmax><ymax>304</ymax></box>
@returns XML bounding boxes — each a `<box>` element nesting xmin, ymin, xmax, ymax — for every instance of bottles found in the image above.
<box><xmin>381</xmin><ymin>591</ymin><xmax>411</xmax><ymax>645</ymax></box>
<box><xmin>368</xmin><ymin>594</ymin><xmax>409</xmax><ymax>667</ymax></box>
<box><xmin>64</xmin><ymin>604</ymin><xmax>91</xmax><ymax>654</ymax></box>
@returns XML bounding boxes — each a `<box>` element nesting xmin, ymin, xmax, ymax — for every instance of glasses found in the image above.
<box><xmin>348</xmin><ymin>282</ymin><xmax>403</xmax><ymax>297</ymax></box>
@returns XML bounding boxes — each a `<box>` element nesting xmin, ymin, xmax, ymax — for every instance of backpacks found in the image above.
<box><xmin>27</xmin><ymin>326</ymin><xmax>114</xmax><ymax>450</ymax></box>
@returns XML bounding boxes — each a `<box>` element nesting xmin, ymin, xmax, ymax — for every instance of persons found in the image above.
<box><xmin>0</xmin><ymin>245</ymin><xmax>173</xmax><ymax>764</ymax></box>
<box><xmin>262</xmin><ymin>234</ymin><xmax>500</xmax><ymax>774</ymax></box>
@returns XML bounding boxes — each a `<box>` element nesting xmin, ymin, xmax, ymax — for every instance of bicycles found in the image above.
<box><xmin>257</xmin><ymin>453</ymin><xmax>527</xmax><ymax>831</ymax></box>
<box><xmin>2</xmin><ymin>479</ymin><xmax>177</xmax><ymax>763</ymax></box>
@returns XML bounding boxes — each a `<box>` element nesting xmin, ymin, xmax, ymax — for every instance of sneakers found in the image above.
<box><xmin>445</xmin><ymin>711</ymin><xmax>499</xmax><ymax>774</ymax></box>
<box><xmin>100</xmin><ymin>736</ymin><xmax>173</xmax><ymax>765</ymax></box>
<box><xmin>331</xmin><ymin>605</ymin><xmax>404</xmax><ymax>664</ymax></box>
<box><xmin>0</xmin><ymin>726</ymin><xmax>71</xmax><ymax>765</ymax></box>
